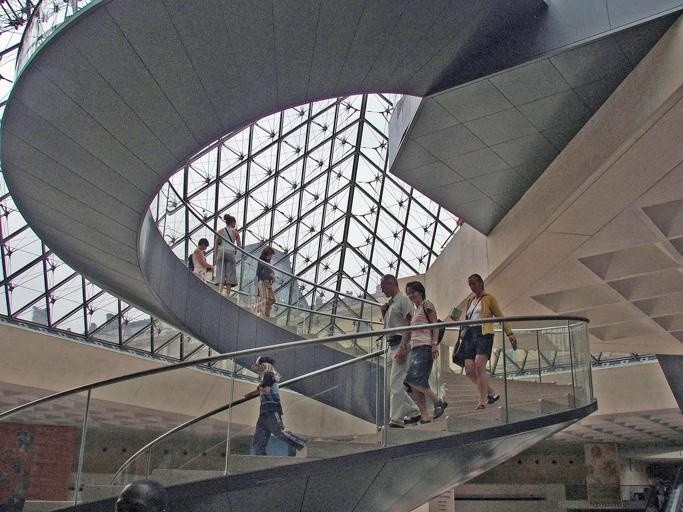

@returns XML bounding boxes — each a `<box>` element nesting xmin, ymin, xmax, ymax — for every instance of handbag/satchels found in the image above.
<box><xmin>453</xmin><ymin>339</ymin><xmax>466</xmax><ymax>367</ymax></box>
<box><xmin>423</xmin><ymin>301</ymin><xmax>445</xmax><ymax>347</ymax></box>
<box><xmin>258</xmin><ymin>262</ymin><xmax>275</xmax><ymax>280</ymax></box>
<box><xmin>186</xmin><ymin>253</ymin><xmax>194</xmax><ymax>271</ymax></box>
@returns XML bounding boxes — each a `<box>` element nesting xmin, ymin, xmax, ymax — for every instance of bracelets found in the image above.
<box><xmin>433</xmin><ymin>342</ymin><xmax>438</xmax><ymax>346</ymax></box>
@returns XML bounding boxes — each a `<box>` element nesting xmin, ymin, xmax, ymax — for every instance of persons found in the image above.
<box><xmin>244</xmin><ymin>355</ymin><xmax>307</xmax><ymax>455</ymax></box>
<box><xmin>452</xmin><ymin>274</ymin><xmax>517</xmax><ymax>410</ymax></box>
<box><xmin>248</xmin><ymin>246</ymin><xmax>276</xmax><ymax>317</ymax></box>
<box><xmin>117</xmin><ymin>481</ymin><xmax>166</xmax><ymax>512</ymax></box>
<box><xmin>403</xmin><ymin>281</ymin><xmax>448</xmax><ymax>426</ymax></box>
<box><xmin>213</xmin><ymin>214</ymin><xmax>240</xmax><ymax>297</ymax></box>
<box><xmin>380</xmin><ymin>274</ymin><xmax>421</xmax><ymax>429</ymax></box>
<box><xmin>192</xmin><ymin>238</ymin><xmax>210</xmax><ymax>281</ymax></box>
<box><xmin>656</xmin><ymin>481</ymin><xmax>667</xmax><ymax>511</ymax></box>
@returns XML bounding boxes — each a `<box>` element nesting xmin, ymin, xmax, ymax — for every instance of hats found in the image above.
<box><xmin>252</xmin><ymin>355</ymin><xmax>275</xmax><ymax>368</ymax></box>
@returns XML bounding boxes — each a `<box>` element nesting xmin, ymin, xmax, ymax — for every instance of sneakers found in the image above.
<box><xmin>404</xmin><ymin>413</ymin><xmax>422</xmax><ymax>426</ymax></box>
<box><xmin>382</xmin><ymin>422</ymin><xmax>406</xmax><ymax>429</ymax></box>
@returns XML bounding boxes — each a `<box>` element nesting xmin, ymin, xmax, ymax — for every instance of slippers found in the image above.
<box><xmin>486</xmin><ymin>393</ymin><xmax>500</xmax><ymax>406</ymax></box>
<box><xmin>474</xmin><ymin>403</ymin><xmax>486</xmax><ymax>410</ymax></box>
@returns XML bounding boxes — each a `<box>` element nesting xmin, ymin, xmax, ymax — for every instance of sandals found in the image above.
<box><xmin>433</xmin><ymin>399</ymin><xmax>448</xmax><ymax>420</ymax></box>
<box><xmin>416</xmin><ymin>417</ymin><xmax>433</xmax><ymax>426</ymax></box>
